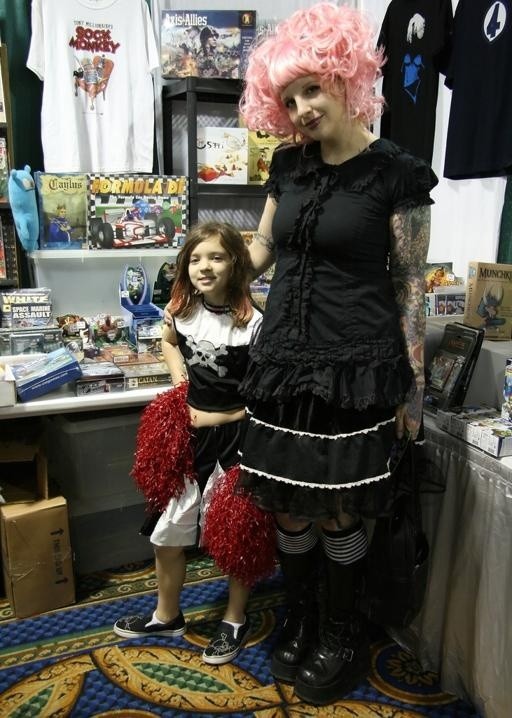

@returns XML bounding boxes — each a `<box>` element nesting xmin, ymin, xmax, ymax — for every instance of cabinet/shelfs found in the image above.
<box><xmin>1</xmin><ymin>63</ymin><xmax>267</xmax><ymax>500</ymax></box>
<box><xmin>373</xmin><ymin>309</ymin><xmax>512</xmax><ymax>715</ymax></box>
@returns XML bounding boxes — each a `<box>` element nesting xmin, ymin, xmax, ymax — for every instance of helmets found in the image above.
<box><xmin>200</xmin><ymin>25</ymin><xmax>219</xmax><ymax>41</ymax></box>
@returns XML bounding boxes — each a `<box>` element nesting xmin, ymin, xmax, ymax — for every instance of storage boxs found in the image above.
<box><xmin>4</xmin><ymin>496</ymin><xmax>78</xmax><ymax>621</ymax></box>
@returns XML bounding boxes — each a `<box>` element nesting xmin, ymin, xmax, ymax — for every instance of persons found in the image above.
<box><xmin>232</xmin><ymin>18</ymin><xmax>437</xmax><ymax>708</ymax></box>
<box><xmin>110</xmin><ymin>221</ymin><xmax>263</xmax><ymax>663</ymax></box>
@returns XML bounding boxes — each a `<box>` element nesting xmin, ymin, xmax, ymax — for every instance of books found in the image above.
<box><xmin>463</xmin><ymin>260</ymin><xmax>512</xmax><ymax>342</ymax></box>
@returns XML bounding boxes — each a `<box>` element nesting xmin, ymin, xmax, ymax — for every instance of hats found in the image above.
<box><xmin>185</xmin><ymin>26</ymin><xmax>199</xmax><ymax>32</ymax></box>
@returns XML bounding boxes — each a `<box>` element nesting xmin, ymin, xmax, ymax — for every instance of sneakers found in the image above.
<box><xmin>113</xmin><ymin>609</ymin><xmax>188</xmax><ymax>638</ymax></box>
<box><xmin>202</xmin><ymin>613</ymin><xmax>252</xmax><ymax>664</ymax></box>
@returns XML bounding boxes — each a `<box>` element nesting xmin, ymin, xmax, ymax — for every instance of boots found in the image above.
<box><xmin>295</xmin><ymin>539</ymin><xmax>380</xmax><ymax>704</ymax></box>
<box><xmin>268</xmin><ymin>538</ymin><xmax>321</xmax><ymax>683</ymax></box>
